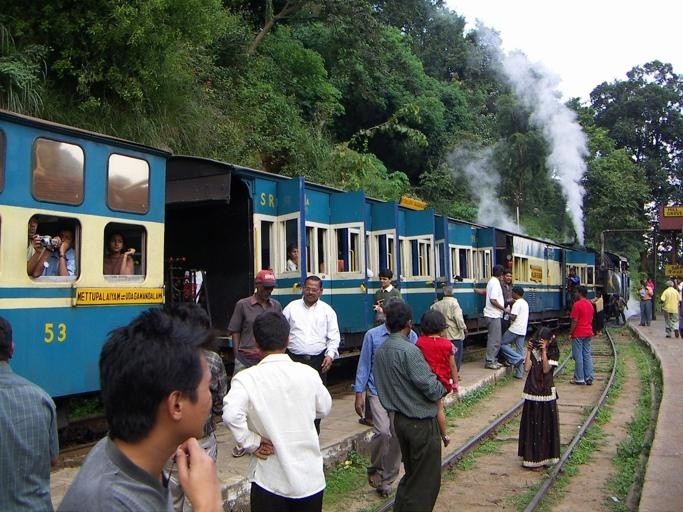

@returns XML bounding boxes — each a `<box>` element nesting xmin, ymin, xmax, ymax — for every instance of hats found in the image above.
<box><xmin>256</xmin><ymin>270</ymin><xmax>276</xmax><ymax>287</ymax></box>
<box><xmin>666</xmin><ymin>281</ymin><xmax>674</xmax><ymax>286</ymax></box>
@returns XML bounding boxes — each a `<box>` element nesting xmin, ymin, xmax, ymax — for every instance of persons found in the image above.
<box><xmin>473</xmin><ymin>268</ymin><xmax>516</xmax><ymax>305</ymax></box>
<box><xmin>568</xmin><ymin>268</ymin><xmax>580</xmax><ymax>287</ymax></box>
<box><xmin>1</xmin><ymin>317</ymin><xmax>59</xmax><ymax>512</ymax></box>
<box><xmin>430</xmin><ymin>285</ymin><xmax>466</xmax><ymax>374</ymax></box>
<box><xmin>414</xmin><ymin>309</ymin><xmax>460</xmax><ymax>447</ymax></box>
<box><xmin>28</xmin><ymin>216</ymin><xmax>76</xmax><ymax>278</ymax></box>
<box><xmin>166</xmin><ymin>301</ymin><xmax>228</xmax><ymax>511</ymax></box>
<box><xmin>282</xmin><ymin>275</ymin><xmax>341</xmax><ymax>436</ymax></box>
<box><xmin>660</xmin><ymin>277</ymin><xmax>683</xmax><ymax>337</ymax></box>
<box><xmin>500</xmin><ymin>286</ymin><xmax>529</xmax><ymax>379</ymax></box>
<box><xmin>354</xmin><ymin>296</ymin><xmax>418</xmax><ymax>499</ymax></box>
<box><xmin>374</xmin><ymin>268</ymin><xmax>403</xmax><ymax>301</ymax></box>
<box><xmin>646</xmin><ymin>278</ymin><xmax>654</xmax><ymax>290</ymax></box>
<box><xmin>103</xmin><ymin>233</ymin><xmax>136</xmax><ymax>275</ymax></box>
<box><xmin>483</xmin><ymin>266</ymin><xmax>510</xmax><ymax>370</ymax></box>
<box><xmin>517</xmin><ymin>326</ymin><xmax>562</xmax><ymax>468</ymax></box>
<box><xmin>285</xmin><ymin>243</ymin><xmax>299</xmax><ymax>271</ymax></box>
<box><xmin>231</xmin><ymin>270</ymin><xmax>282</xmax><ymax>457</ymax></box>
<box><xmin>569</xmin><ymin>286</ymin><xmax>595</xmax><ymax>385</ymax></box>
<box><xmin>638</xmin><ymin>279</ymin><xmax>653</xmax><ymax>326</ymax></box>
<box><xmin>58</xmin><ymin>307</ymin><xmax>223</xmax><ymax>512</ymax></box>
<box><xmin>374</xmin><ymin>301</ymin><xmax>448</xmax><ymax>512</ymax></box>
<box><xmin>222</xmin><ymin>311</ymin><xmax>332</xmax><ymax>511</ymax></box>
<box><xmin>612</xmin><ymin>294</ymin><xmax>628</xmax><ymax>321</ymax></box>
<box><xmin>591</xmin><ymin>289</ymin><xmax>604</xmax><ymax>336</ymax></box>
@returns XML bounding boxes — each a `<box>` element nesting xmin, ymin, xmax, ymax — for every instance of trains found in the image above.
<box><xmin>2</xmin><ymin>110</ymin><xmax>628</xmax><ymax>416</ymax></box>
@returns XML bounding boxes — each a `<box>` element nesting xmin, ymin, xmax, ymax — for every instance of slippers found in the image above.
<box><xmin>232</xmin><ymin>445</ymin><xmax>246</xmax><ymax>456</ymax></box>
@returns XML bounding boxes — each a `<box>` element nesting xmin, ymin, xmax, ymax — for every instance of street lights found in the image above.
<box><xmin>648</xmin><ymin>220</ymin><xmax>658</xmax><ymax>321</ymax></box>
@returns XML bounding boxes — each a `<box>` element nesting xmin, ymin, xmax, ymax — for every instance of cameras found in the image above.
<box><xmin>37</xmin><ymin>235</ymin><xmax>59</xmax><ymax>247</ymax></box>
<box><xmin>528</xmin><ymin>339</ymin><xmax>542</xmax><ymax>349</ymax></box>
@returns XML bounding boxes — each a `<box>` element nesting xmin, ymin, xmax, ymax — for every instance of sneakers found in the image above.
<box><xmin>486</xmin><ymin>357</ymin><xmax>524</xmax><ymax>378</ymax></box>
